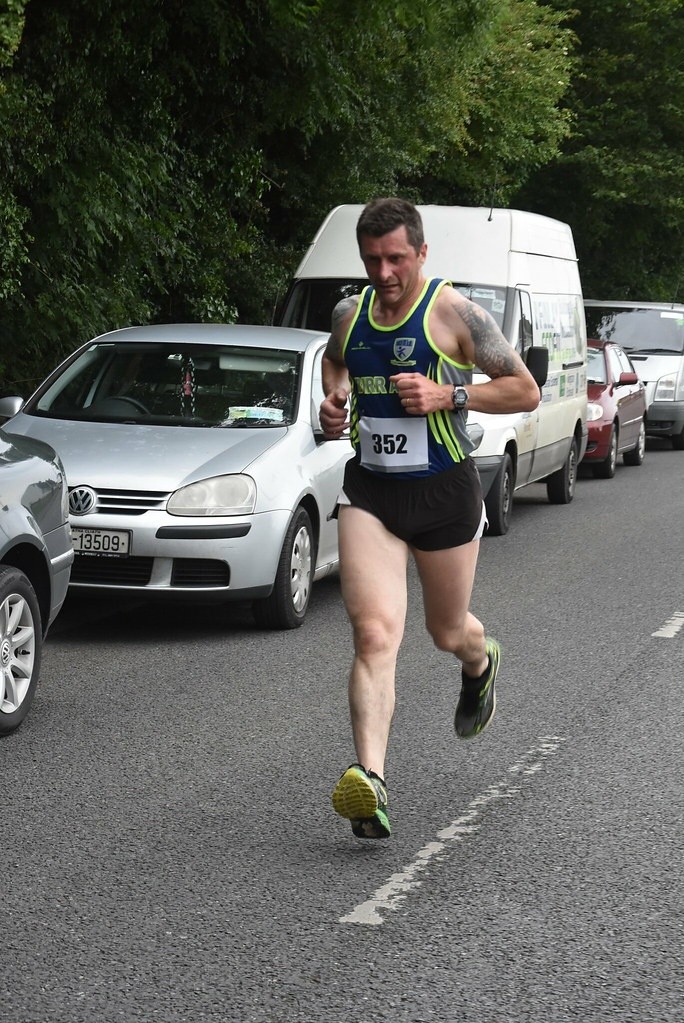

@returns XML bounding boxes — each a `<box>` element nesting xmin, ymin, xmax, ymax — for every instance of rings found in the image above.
<box><xmin>406</xmin><ymin>397</ymin><xmax>410</xmax><ymax>406</ymax></box>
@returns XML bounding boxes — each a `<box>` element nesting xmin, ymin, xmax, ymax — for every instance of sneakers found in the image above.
<box><xmin>454</xmin><ymin>636</ymin><xmax>500</xmax><ymax>737</ymax></box>
<box><xmin>332</xmin><ymin>763</ymin><xmax>391</xmax><ymax>840</ymax></box>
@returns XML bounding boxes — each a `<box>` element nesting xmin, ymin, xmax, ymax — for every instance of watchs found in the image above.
<box><xmin>451</xmin><ymin>383</ymin><xmax>470</xmax><ymax>412</ymax></box>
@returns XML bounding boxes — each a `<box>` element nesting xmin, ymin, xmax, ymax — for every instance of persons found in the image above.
<box><xmin>317</xmin><ymin>197</ymin><xmax>541</xmax><ymax>837</ymax></box>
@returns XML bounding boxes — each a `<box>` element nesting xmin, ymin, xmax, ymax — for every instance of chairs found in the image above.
<box><xmin>121</xmin><ymin>353</ymin><xmax>274</xmax><ymax>422</ymax></box>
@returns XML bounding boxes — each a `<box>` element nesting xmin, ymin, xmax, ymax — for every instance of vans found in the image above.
<box><xmin>279</xmin><ymin>202</ymin><xmax>587</xmax><ymax>535</ymax></box>
<box><xmin>584</xmin><ymin>299</ymin><xmax>684</xmax><ymax>451</ymax></box>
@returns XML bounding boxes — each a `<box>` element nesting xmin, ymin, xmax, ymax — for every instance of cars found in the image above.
<box><xmin>0</xmin><ymin>430</ymin><xmax>73</xmax><ymax>735</ymax></box>
<box><xmin>0</xmin><ymin>323</ymin><xmax>355</xmax><ymax>628</ymax></box>
<box><xmin>587</xmin><ymin>338</ymin><xmax>648</xmax><ymax>477</ymax></box>
